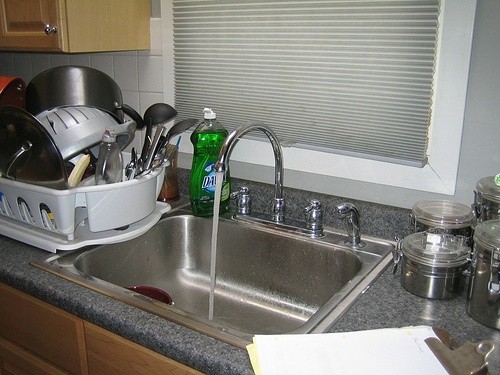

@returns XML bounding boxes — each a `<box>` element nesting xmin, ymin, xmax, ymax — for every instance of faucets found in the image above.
<box><xmin>215</xmin><ymin>121</ymin><xmax>286</xmax><ymax>224</ymax></box>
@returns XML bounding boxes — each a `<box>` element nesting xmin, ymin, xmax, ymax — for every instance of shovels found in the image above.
<box><xmin>150</xmin><ymin>118</ymin><xmax>200</xmax><ymax>167</ymax></box>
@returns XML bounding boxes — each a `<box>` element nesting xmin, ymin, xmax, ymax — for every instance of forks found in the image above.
<box><xmin>166</xmin><ymin>143</ymin><xmax>176</xmax><ymax>157</ymax></box>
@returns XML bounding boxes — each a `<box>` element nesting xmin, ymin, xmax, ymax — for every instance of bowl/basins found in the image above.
<box><xmin>1</xmin><ymin>75</ymin><xmax>26</xmax><ymax>108</ymax></box>
<box><xmin>126</xmin><ymin>286</ymin><xmax>173</xmax><ymax>307</ymax></box>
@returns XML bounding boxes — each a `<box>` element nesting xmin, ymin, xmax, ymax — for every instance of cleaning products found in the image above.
<box><xmin>190</xmin><ymin>109</ymin><xmax>230</xmax><ymax>218</ymax></box>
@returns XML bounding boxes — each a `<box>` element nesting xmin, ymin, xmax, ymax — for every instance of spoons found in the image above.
<box><xmin>141</xmin><ymin>102</ymin><xmax>176</xmax><ymax>164</ymax></box>
<box><xmin>154</xmin><ymin>119</ymin><xmax>198</xmax><ymax>158</ymax></box>
<box><xmin>130</xmin><ymin>145</ymin><xmax>139</xmax><ymax>180</ymax></box>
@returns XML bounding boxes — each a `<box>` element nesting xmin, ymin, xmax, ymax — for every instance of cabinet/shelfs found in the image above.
<box><xmin>0</xmin><ymin>0</ymin><xmax>152</xmax><ymax>53</ymax></box>
<box><xmin>86</xmin><ymin>317</ymin><xmax>207</xmax><ymax>375</ymax></box>
<box><xmin>1</xmin><ymin>280</ymin><xmax>86</xmax><ymax>374</ymax></box>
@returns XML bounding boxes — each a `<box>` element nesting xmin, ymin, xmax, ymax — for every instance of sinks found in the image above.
<box><xmin>30</xmin><ymin>201</ymin><xmax>395</xmax><ymax>351</ymax></box>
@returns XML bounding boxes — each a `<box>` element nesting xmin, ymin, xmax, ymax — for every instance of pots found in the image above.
<box><xmin>24</xmin><ymin>65</ymin><xmax>144</xmax><ymax>130</ymax></box>
